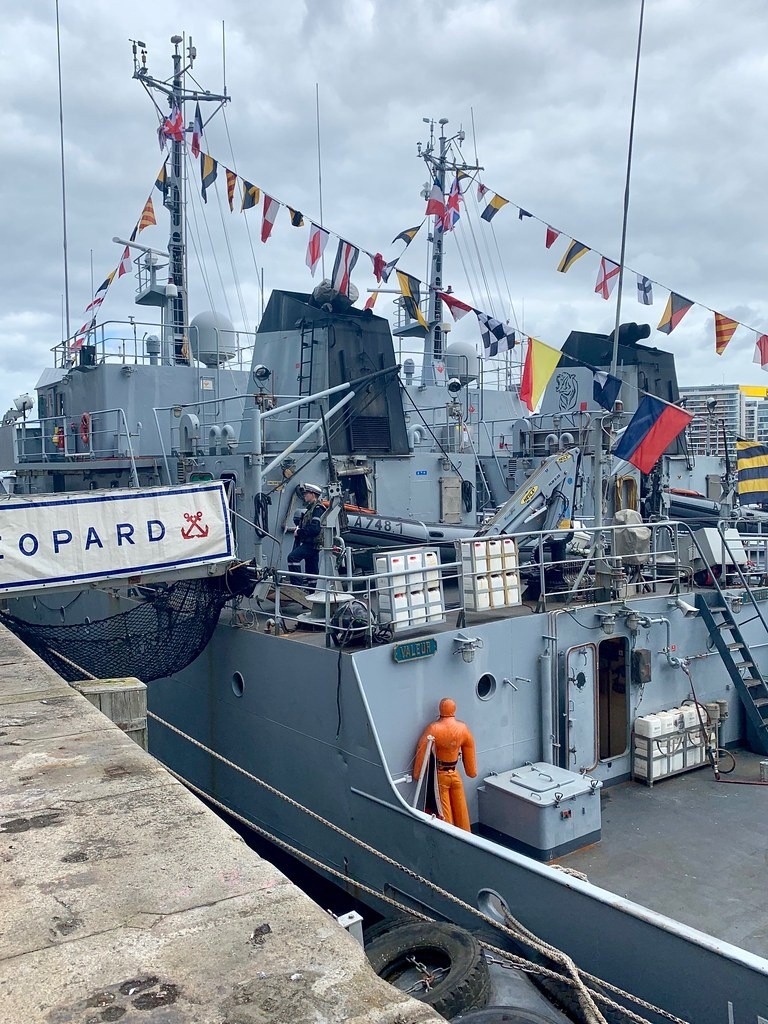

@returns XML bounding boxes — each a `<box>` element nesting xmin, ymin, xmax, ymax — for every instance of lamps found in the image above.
<box><xmin>453</xmin><ymin>634</ymin><xmax>484</xmax><ymax>664</ymax></box>
<box><xmin>727</xmin><ymin>595</ymin><xmax>743</xmax><ymax>615</ymax></box>
<box><xmin>594</xmin><ymin>607</ymin><xmax>616</xmax><ymax>635</ymax></box>
<box><xmin>707</xmin><ymin>397</ymin><xmax>718</xmax><ymax>419</ymax></box>
<box><xmin>14</xmin><ymin>394</ymin><xmax>33</xmax><ymax>421</ymax></box>
<box><xmin>185</xmin><ymin>457</ymin><xmax>203</xmax><ymax>473</ymax></box>
<box><xmin>120</xmin><ymin>365</ymin><xmax>133</xmax><ymax>378</ymax></box>
<box><xmin>623</xmin><ymin>614</ymin><xmax>638</xmax><ymax>632</ymax></box>
<box><xmin>254</xmin><ymin>364</ymin><xmax>272</xmax><ymax>393</ymax></box>
<box><xmin>62</xmin><ymin>374</ymin><xmax>72</xmax><ymax>386</ymax></box>
<box><xmin>440</xmin><ymin>456</ymin><xmax>452</xmax><ymax>472</ymax></box>
<box><xmin>171</xmin><ymin>404</ymin><xmax>183</xmax><ymax>419</ymax></box>
<box><xmin>448</xmin><ymin>377</ymin><xmax>461</xmax><ymax>406</ymax></box>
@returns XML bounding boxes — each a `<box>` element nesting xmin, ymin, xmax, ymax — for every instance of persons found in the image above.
<box><xmin>287</xmin><ymin>483</ymin><xmax>327</xmax><ymax>594</ymax></box>
<box><xmin>615</xmin><ymin>400</ymin><xmax>623</xmax><ymax>411</ymax></box>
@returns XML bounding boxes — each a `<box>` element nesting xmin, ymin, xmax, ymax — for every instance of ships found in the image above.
<box><xmin>0</xmin><ymin>1</ymin><xmax>768</xmax><ymax>1024</ymax></box>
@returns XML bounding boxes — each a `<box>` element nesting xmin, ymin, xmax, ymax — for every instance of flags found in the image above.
<box><xmin>64</xmin><ymin>103</ymin><xmax>766</xmax><ymax>502</ymax></box>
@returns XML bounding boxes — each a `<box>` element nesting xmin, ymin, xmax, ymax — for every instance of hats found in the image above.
<box><xmin>302</xmin><ymin>482</ymin><xmax>323</xmax><ymax>495</ymax></box>
